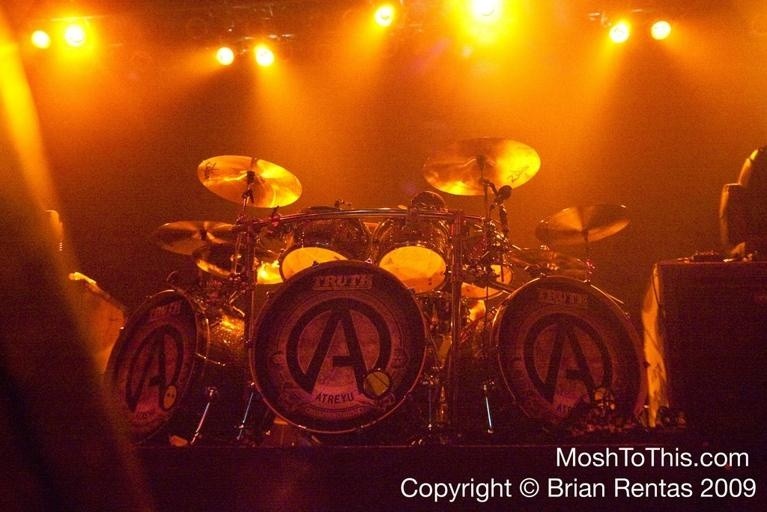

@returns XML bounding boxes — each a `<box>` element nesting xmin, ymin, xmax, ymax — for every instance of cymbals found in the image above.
<box><xmin>193</xmin><ymin>242</ymin><xmax>283</xmax><ymax>285</ymax></box>
<box><xmin>504</xmin><ymin>248</ymin><xmax>600</xmax><ymax>279</ymax></box>
<box><xmin>535</xmin><ymin>203</ymin><xmax>631</xmax><ymax>246</ymax></box>
<box><xmin>423</xmin><ymin>137</ymin><xmax>540</xmax><ymax>196</ymax></box>
<box><xmin>197</xmin><ymin>154</ymin><xmax>302</xmax><ymax>209</ymax></box>
<box><xmin>154</xmin><ymin>220</ymin><xmax>284</xmax><ymax>263</ymax></box>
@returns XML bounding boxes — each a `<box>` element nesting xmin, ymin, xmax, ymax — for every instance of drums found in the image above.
<box><xmin>443</xmin><ymin>275</ymin><xmax>649</xmax><ymax>442</ymax></box>
<box><xmin>369</xmin><ymin>205</ymin><xmax>452</xmax><ymax>296</ymax></box>
<box><xmin>246</xmin><ymin>260</ymin><xmax>442</xmax><ymax>435</ymax></box>
<box><xmin>101</xmin><ymin>287</ymin><xmax>271</xmax><ymax>444</ymax></box>
<box><xmin>457</xmin><ymin>216</ymin><xmax>512</xmax><ymax>301</ymax></box>
<box><xmin>279</xmin><ymin>205</ymin><xmax>371</xmax><ymax>280</ymax></box>
